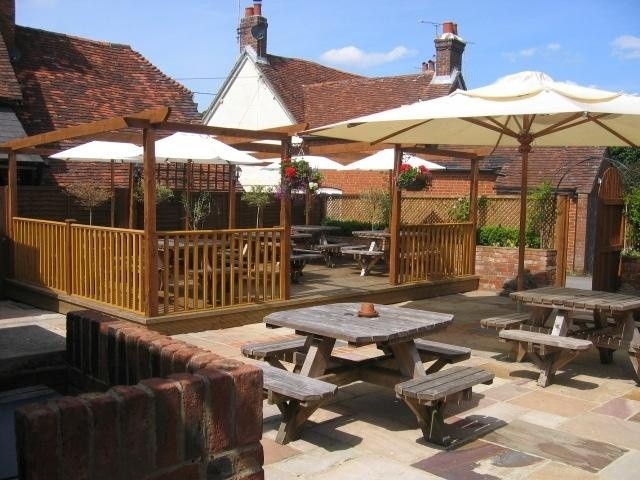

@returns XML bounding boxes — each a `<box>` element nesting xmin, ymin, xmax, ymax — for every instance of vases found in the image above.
<box><xmin>405</xmin><ymin>178</ymin><xmax>426</xmax><ymax>191</ymax></box>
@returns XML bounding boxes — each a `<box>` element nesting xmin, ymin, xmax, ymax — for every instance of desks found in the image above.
<box><xmin>509</xmin><ymin>287</ymin><xmax>639</xmax><ymax>388</ymax></box>
<box><xmin>261</xmin><ymin>301</ymin><xmax>455</xmax><ymax>445</ymax></box>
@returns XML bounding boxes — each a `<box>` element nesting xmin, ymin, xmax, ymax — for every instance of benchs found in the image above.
<box><xmin>252</xmin><ymin>361</ymin><xmax>338</xmax><ymax>446</ymax></box>
<box><xmin>480</xmin><ymin>313</ymin><xmax>640</xmax><ymax>388</ymax></box>
<box><xmin>394</xmin><ymin>365</ymin><xmax>508</xmax><ymax>451</ymax></box>
<box><xmin>240</xmin><ymin>336</ymin><xmax>307</xmax><ymax>371</ymax></box>
<box><xmin>413</xmin><ymin>337</ymin><xmax>471</xmax><ymax>373</ymax></box>
<box><xmin>157</xmin><ymin>225</ymin><xmax>441</xmax><ymax>305</ymax></box>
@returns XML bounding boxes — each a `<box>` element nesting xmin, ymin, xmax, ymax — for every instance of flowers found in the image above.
<box><xmin>392</xmin><ymin>162</ymin><xmax>434</xmax><ymax>191</ymax></box>
<box><xmin>273</xmin><ymin>149</ymin><xmax>324</xmax><ymax>200</ymax></box>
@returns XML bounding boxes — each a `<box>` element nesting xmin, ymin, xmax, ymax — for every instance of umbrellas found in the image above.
<box><xmin>296</xmin><ymin>69</ymin><xmax>640</xmax><ymax>313</ymax></box>
<box><xmin>45</xmin><ymin>138</ymin><xmax>143</xmax><ymax>230</ymax></box>
<box><xmin>125</xmin><ymin>131</ymin><xmax>267</xmax><ymax>230</ymax></box>
<box><xmin>265</xmin><ymin>156</ymin><xmax>349</xmax><ymax>176</ymax></box>
<box><xmin>337</xmin><ymin>143</ymin><xmax>449</xmax><ymax>196</ymax></box>
